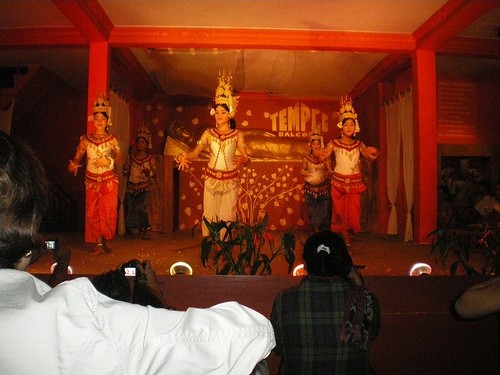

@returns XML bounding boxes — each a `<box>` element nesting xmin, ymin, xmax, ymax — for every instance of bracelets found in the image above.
<box><xmin>107</xmin><ymin>157</ymin><xmax>114</xmax><ymax>168</ymax></box>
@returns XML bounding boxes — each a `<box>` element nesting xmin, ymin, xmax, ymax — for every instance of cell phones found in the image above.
<box><xmin>122</xmin><ymin>264</ymin><xmax>137</xmax><ymax>284</ymax></box>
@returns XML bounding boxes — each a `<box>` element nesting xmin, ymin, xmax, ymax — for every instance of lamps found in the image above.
<box><xmin>293</xmin><ymin>264</ymin><xmax>309</xmax><ymax>275</ymax></box>
<box><xmin>51</xmin><ymin>262</ymin><xmax>72</xmax><ymax>274</ymax></box>
<box><xmin>170</xmin><ymin>262</ymin><xmax>193</xmax><ymax>276</ymax></box>
<box><xmin>409</xmin><ymin>263</ymin><xmax>432</xmax><ymax>277</ymax></box>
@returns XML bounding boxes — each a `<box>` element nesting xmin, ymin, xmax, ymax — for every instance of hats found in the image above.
<box><xmin>91</xmin><ymin>96</ymin><xmax>112</xmax><ymax>116</ymax></box>
<box><xmin>214</xmin><ymin>68</ymin><xmax>239</xmax><ymax>119</ymax></box>
<box><xmin>137</xmin><ymin>126</ymin><xmax>150</xmax><ymax>139</ymax></box>
<box><xmin>309</xmin><ymin>129</ymin><xmax>323</xmax><ymax>147</ymax></box>
<box><xmin>338</xmin><ymin>95</ymin><xmax>360</xmax><ymax>133</ymax></box>
<box><xmin>303</xmin><ymin>231</ymin><xmax>352</xmax><ymax>277</ymax></box>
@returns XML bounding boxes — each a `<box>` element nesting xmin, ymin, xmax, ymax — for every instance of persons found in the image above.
<box><xmin>123</xmin><ymin>127</ymin><xmax>156</xmax><ymax>234</ymax></box>
<box><xmin>300</xmin><ymin>139</ymin><xmax>332</xmax><ymax>232</ymax></box>
<box><xmin>178</xmin><ymin>103</ymin><xmax>250</xmax><ymax>260</ymax></box>
<box><xmin>0</xmin><ymin>133</ymin><xmax>276</xmax><ymax>374</ymax></box>
<box><xmin>454</xmin><ymin>276</ymin><xmax>500</xmax><ymax>319</ymax></box>
<box><xmin>311</xmin><ymin>118</ymin><xmax>378</xmax><ymax>249</ymax></box>
<box><xmin>68</xmin><ymin>98</ymin><xmax>121</xmax><ymax>254</ymax></box>
<box><xmin>271</xmin><ymin>231</ymin><xmax>380</xmax><ymax>374</ymax></box>
